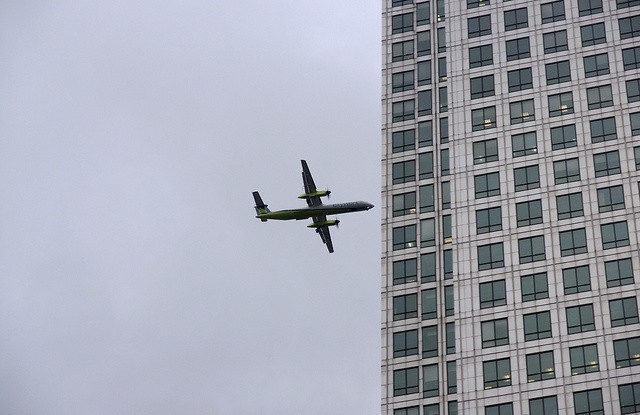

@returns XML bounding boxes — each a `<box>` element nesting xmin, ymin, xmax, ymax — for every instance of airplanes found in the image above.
<box><xmin>252</xmin><ymin>160</ymin><xmax>374</xmax><ymax>253</ymax></box>
